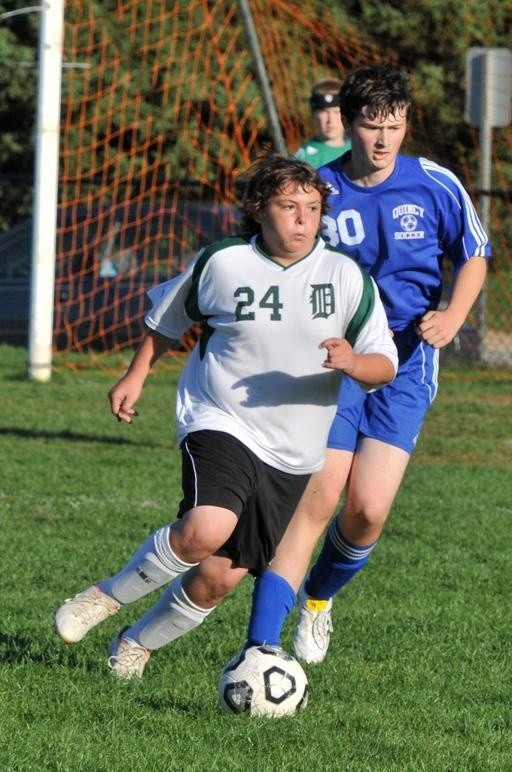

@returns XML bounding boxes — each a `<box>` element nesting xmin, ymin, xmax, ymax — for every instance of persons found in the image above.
<box><xmin>54</xmin><ymin>156</ymin><xmax>401</xmax><ymax>682</ymax></box>
<box><xmin>241</xmin><ymin>57</ymin><xmax>494</xmax><ymax>667</ymax></box>
<box><xmin>286</xmin><ymin>77</ymin><xmax>357</xmax><ymax>175</ymax></box>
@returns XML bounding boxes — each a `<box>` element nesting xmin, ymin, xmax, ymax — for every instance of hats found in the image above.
<box><xmin>0</xmin><ymin>198</ymin><xmax>260</xmax><ymax>355</ymax></box>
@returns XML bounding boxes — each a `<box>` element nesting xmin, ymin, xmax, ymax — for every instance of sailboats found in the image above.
<box><xmin>221</xmin><ymin>646</ymin><xmax>309</xmax><ymax>718</ymax></box>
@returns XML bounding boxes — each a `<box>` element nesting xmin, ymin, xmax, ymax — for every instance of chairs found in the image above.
<box><xmin>292</xmin><ymin>574</ymin><xmax>334</xmax><ymax>663</ymax></box>
<box><xmin>53</xmin><ymin>581</ymin><xmax>123</xmax><ymax>647</ymax></box>
<box><xmin>107</xmin><ymin>623</ymin><xmax>154</xmax><ymax>680</ymax></box>
